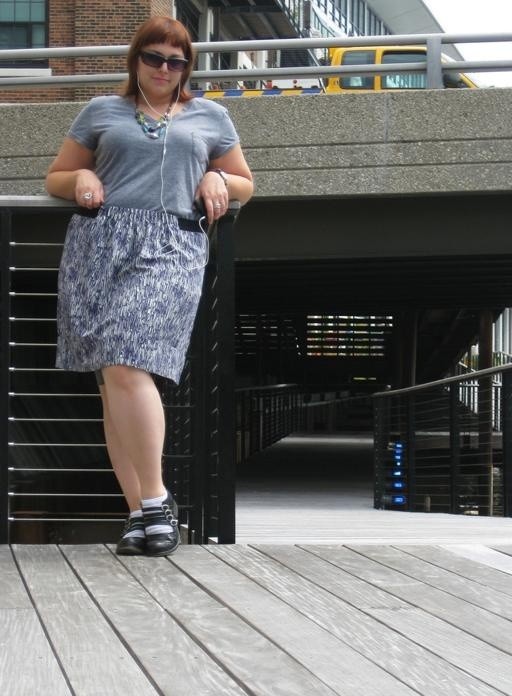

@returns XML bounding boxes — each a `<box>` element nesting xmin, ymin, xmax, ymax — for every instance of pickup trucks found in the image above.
<box><xmin>184</xmin><ymin>45</ymin><xmax>482</xmax><ymax>98</ymax></box>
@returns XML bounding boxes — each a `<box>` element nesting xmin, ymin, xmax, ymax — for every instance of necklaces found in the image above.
<box><xmin>135</xmin><ymin>92</ymin><xmax>175</xmax><ymax>138</ymax></box>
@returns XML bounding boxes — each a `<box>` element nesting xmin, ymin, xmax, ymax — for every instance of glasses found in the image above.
<box><xmin>136</xmin><ymin>50</ymin><xmax>190</xmax><ymax>73</ymax></box>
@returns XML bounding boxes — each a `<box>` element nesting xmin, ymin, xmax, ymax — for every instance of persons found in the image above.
<box><xmin>46</xmin><ymin>16</ymin><xmax>254</xmax><ymax>558</ymax></box>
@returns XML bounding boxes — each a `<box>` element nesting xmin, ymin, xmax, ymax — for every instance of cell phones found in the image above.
<box><xmin>193</xmin><ymin>197</ymin><xmax>208</xmax><ymax>220</ymax></box>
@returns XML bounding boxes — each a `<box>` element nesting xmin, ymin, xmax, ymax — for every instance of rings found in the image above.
<box><xmin>84</xmin><ymin>192</ymin><xmax>93</xmax><ymax>199</ymax></box>
<box><xmin>213</xmin><ymin>202</ymin><xmax>221</xmax><ymax>209</ymax></box>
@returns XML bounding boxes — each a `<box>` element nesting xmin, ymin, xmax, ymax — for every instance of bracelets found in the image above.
<box><xmin>207</xmin><ymin>168</ymin><xmax>228</xmax><ymax>186</ymax></box>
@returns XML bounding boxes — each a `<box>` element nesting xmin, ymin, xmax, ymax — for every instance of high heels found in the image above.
<box><xmin>141</xmin><ymin>490</ymin><xmax>180</xmax><ymax>557</ymax></box>
<box><xmin>116</xmin><ymin>516</ymin><xmax>147</xmax><ymax>555</ymax></box>
